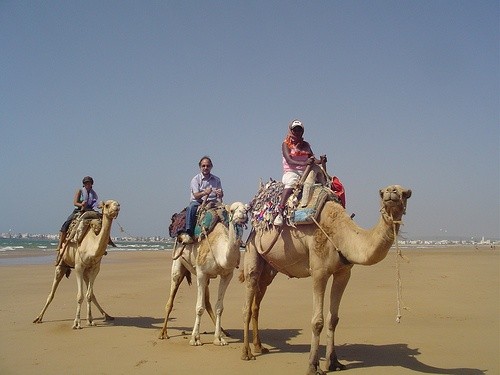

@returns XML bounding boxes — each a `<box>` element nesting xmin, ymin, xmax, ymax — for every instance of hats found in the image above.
<box><xmin>82</xmin><ymin>176</ymin><xmax>93</xmax><ymax>185</ymax></box>
<box><xmin>291</xmin><ymin>121</ymin><xmax>303</xmax><ymax>129</ymax></box>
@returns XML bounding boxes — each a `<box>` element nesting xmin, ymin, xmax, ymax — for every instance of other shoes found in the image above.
<box><xmin>182</xmin><ymin>234</ymin><xmax>194</xmax><ymax>245</ymax></box>
<box><xmin>274</xmin><ymin>214</ymin><xmax>283</xmax><ymax>226</ymax></box>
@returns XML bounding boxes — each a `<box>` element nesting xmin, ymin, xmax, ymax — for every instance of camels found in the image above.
<box><xmin>159</xmin><ymin>201</ymin><xmax>248</xmax><ymax>346</ymax></box>
<box><xmin>32</xmin><ymin>200</ymin><xmax>120</xmax><ymax>329</ymax></box>
<box><xmin>238</xmin><ymin>184</ymin><xmax>413</xmax><ymax>375</ymax></box>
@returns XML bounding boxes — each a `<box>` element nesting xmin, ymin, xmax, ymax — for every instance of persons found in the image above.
<box><xmin>273</xmin><ymin>119</ymin><xmax>327</xmax><ymax>226</ymax></box>
<box><xmin>181</xmin><ymin>155</ymin><xmax>246</xmax><ymax>248</ymax></box>
<box><xmin>55</xmin><ymin>176</ymin><xmax>117</xmax><ymax>255</ymax></box>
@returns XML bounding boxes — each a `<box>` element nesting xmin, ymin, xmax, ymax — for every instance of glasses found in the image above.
<box><xmin>202</xmin><ymin>165</ymin><xmax>211</xmax><ymax>168</ymax></box>
